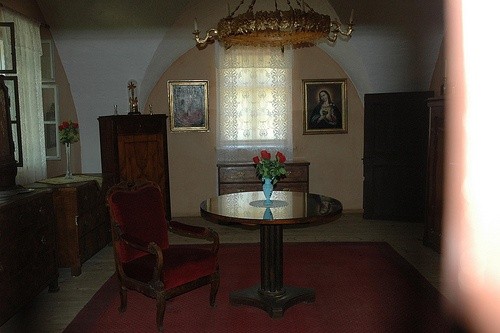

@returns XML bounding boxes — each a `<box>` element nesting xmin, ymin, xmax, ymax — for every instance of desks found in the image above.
<box><xmin>199</xmin><ymin>190</ymin><xmax>343</xmax><ymax>319</ymax></box>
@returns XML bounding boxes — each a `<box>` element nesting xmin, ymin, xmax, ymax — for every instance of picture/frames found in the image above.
<box><xmin>167</xmin><ymin>79</ymin><xmax>211</xmax><ymax>133</ymax></box>
<box><xmin>301</xmin><ymin>78</ymin><xmax>348</xmax><ymax>136</ymax></box>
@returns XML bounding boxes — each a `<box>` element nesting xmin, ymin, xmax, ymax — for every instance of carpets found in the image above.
<box><xmin>60</xmin><ymin>242</ymin><xmax>478</xmax><ymax>333</ymax></box>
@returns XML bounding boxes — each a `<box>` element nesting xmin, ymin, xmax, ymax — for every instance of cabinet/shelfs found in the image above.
<box><xmin>0</xmin><ymin>185</ymin><xmax>60</xmax><ymax>333</ymax></box>
<box><xmin>35</xmin><ymin>172</ymin><xmax>108</xmax><ymax>277</ymax></box>
<box><xmin>423</xmin><ymin>95</ymin><xmax>445</xmax><ymax>254</ymax></box>
<box><xmin>363</xmin><ymin>90</ymin><xmax>434</xmax><ymax>225</ymax></box>
<box><xmin>97</xmin><ymin>113</ymin><xmax>172</xmax><ymax>224</ymax></box>
<box><xmin>217</xmin><ymin>160</ymin><xmax>310</xmax><ymax>196</ymax></box>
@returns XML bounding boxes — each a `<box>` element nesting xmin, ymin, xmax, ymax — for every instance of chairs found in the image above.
<box><xmin>104</xmin><ymin>174</ymin><xmax>223</xmax><ymax>333</ymax></box>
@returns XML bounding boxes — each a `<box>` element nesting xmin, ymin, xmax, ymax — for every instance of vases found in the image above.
<box><xmin>261</xmin><ymin>176</ymin><xmax>275</xmax><ymax>204</ymax></box>
<box><xmin>64</xmin><ymin>143</ymin><xmax>74</xmax><ymax>179</ymax></box>
<box><xmin>263</xmin><ymin>207</ymin><xmax>273</xmax><ymax>220</ymax></box>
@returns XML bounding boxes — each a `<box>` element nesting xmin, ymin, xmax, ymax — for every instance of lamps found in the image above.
<box><xmin>191</xmin><ymin>0</ymin><xmax>357</xmax><ymax>54</ymax></box>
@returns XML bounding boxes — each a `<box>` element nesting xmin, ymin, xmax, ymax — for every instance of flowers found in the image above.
<box><xmin>58</xmin><ymin>120</ymin><xmax>80</xmax><ymax>148</ymax></box>
<box><xmin>251</xmin><ymin>150</ymin><xmax>292</xmax><ymax>185</ymax></box>
